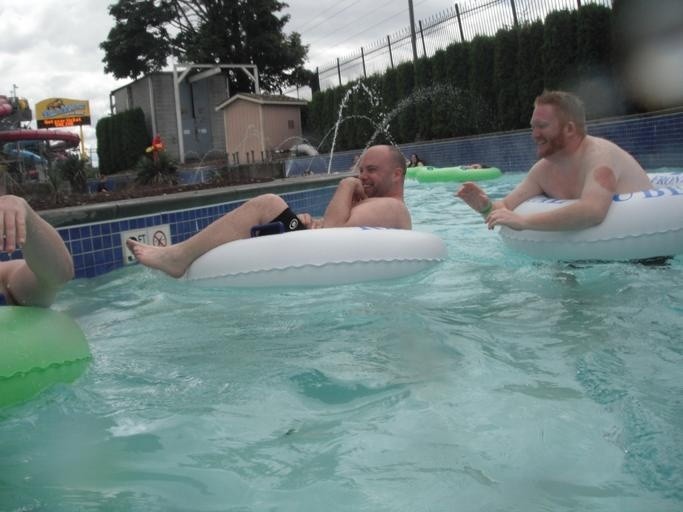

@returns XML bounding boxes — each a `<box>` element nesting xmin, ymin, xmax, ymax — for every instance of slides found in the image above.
<box><xmin>0</xmin><ymin>94</ymin><xmax>81</xmax><ymax>178</ymax></box>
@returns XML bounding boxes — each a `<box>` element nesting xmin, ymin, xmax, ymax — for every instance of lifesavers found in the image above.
<box><xmin>498</xmin><ymin>171</ymin><xmax>683</xmax><ymax>260</ymax></box>
<box><xmin>1</xmin><ymin>305</ymin><xmax>90</xmax><ymax>421</ymax></box>
<box><xmin>177</xmin><ymin>225</ymin><xmax>445</xmax><ymax>287</ymax></box>
<box><xmin>402</xmin><ymin>166</ymin><xmax>502</xmax><ymax>183</ymax></box>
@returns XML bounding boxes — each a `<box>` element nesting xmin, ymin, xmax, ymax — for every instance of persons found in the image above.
<box><xmin>127</xmin><ymin>144</ymin><xmax>412</xmax><ymax>278</ymax></box>
<box><xmin>453</xmin><ymin>87</ymin><xmax>673</xmax><ymax>266</ymax></box>
<box><xmin>350</xmin><ymin>152</ymin><xmax>361</xmax><ymax>172</ymax></box>
<box><xmin>409</xmin><ymin>154</ymin><xmax>426</xmax><ymax>167</ymax></box>
<box><xmin>96</xmin><ymin>175</ymin><xmax>109</xmax><ymax>192</ymax></box>
<box><xmin>0</xmin><ymin>194</ymin><xmax>75</xmax><ymax>307</ymax></box>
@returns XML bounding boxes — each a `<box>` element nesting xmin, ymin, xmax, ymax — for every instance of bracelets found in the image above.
<box><xmin>479</xmin><ymin>200</ymin><xmax>492</xmax><ymax>214</ymax></box>
